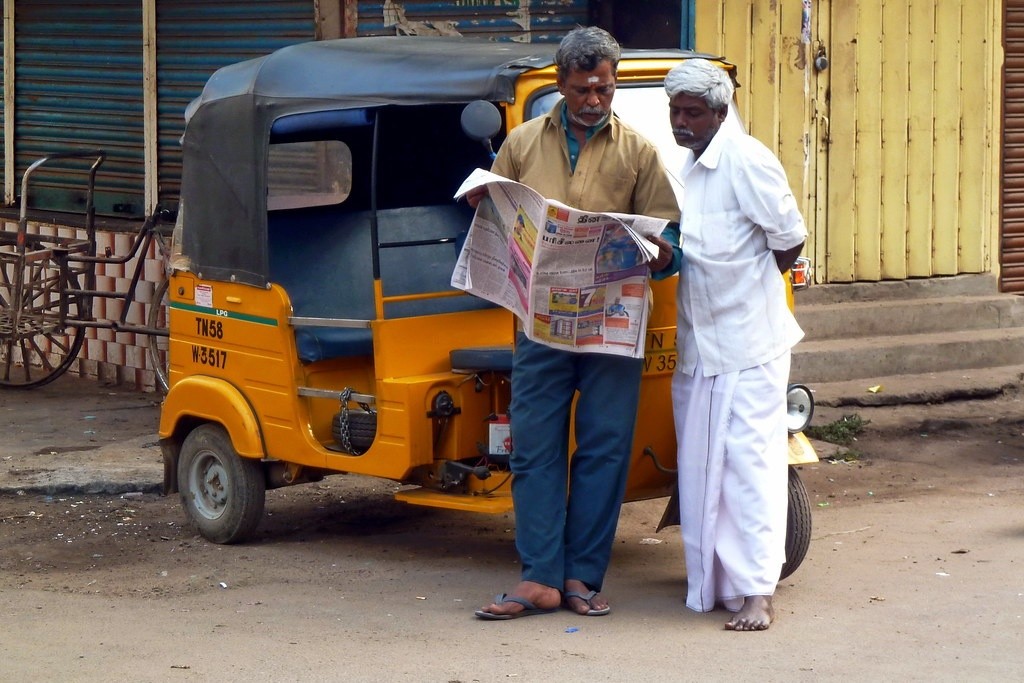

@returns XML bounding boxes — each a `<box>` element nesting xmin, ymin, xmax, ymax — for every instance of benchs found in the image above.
<box><xmin>272</xmin><ymin>200</ymin><xmax>500</xmax><ymax>365</ymax></box>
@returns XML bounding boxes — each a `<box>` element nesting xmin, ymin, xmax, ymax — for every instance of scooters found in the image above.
<box><xmin>158</xmin><ymin>36</ymin><xmax>819</xmax><ymax>585</ymax></box>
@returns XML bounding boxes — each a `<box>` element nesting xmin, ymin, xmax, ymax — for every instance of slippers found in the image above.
<box><xmin>475</xmin><ymin>593</ymin><xmax>561</xmax><ymax>619</ymax></box>
<box><xmin>565</xmin><ymin>590</ymin><xmax>610</xmax><ymax>615</ymax></box>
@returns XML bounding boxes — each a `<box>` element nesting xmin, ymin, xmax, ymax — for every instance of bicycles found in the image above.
<box><xmin>0</xmin><ymin>150</ymin><xmax>177</xmax><ymax>397</ymax></box>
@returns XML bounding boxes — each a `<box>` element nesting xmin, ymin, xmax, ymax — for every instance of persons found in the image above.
<box><xmin>597</xmin><ymin>225</ymin><xmax>639</xmax><ymax>272</ymax></box>
<box><xmin>606</xmin><ymin>296</ymin><xmax>626</xmax><ymax>318</ymax></box>
<box><xmin>514</xmin><ymin>214</ymin><xmax>525</xmax><ymax>242</ymax></box>
<box><xmin>664</xmin><ymin>58</ymin><xmax>807</xmax><ymax>630</ymax></box>
<box><xmin>465</xmin><ymin>26</ymin><xmax>685</xmax><ymax>620</ymax></box>
